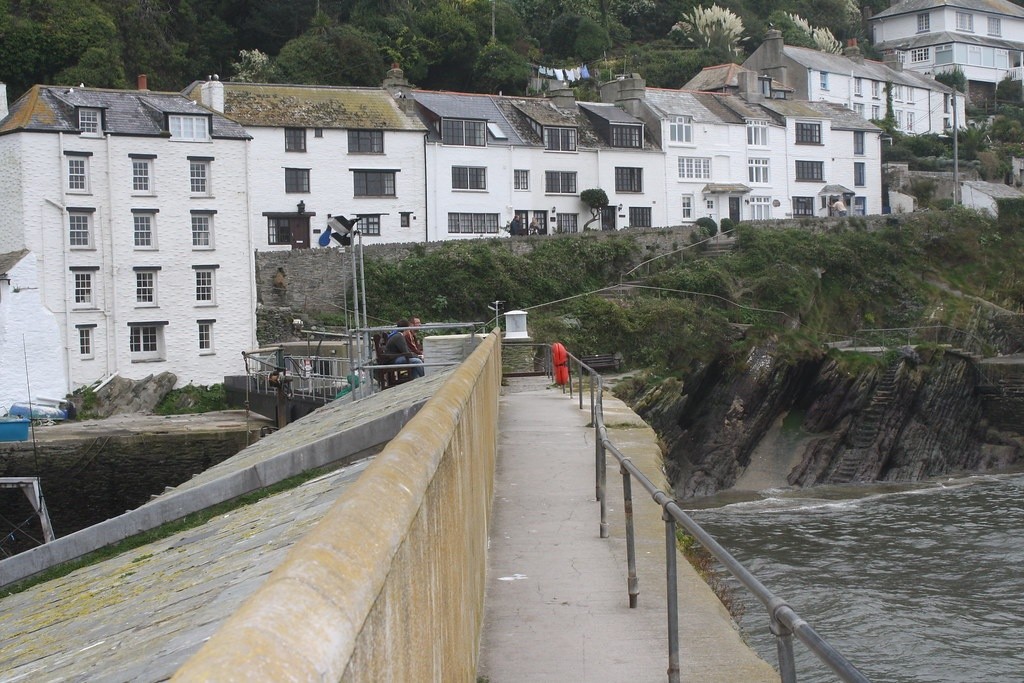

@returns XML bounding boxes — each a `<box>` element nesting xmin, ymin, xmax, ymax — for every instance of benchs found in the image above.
<box><xmin>579</xmin><ymin>352</ymin><xmax>621</xmax><ymax>374</ymax></box>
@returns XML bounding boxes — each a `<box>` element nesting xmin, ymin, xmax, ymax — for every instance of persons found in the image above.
<box><xmin>530</xmin><ymin>217</ymin><xmax>539</xmax><ymax>236</ymax></box>
<box><xmin>510</xmin><ymin>215</ymin><xmax>521</xmax><ymax>235</ymax></box>
<box><xmin>827</xmin><ymin>198</ymin><xmax>847</xmax><ymax>217</ymax></box>
<box><xmin>385</xmin><ymin>319</ymin><xmax>424</xmax><ymax>380</ymax></box>
<box><xmin>403</xmin><ymin>316</ymin><xmax>424</xmax><ymax>363</ymax></box>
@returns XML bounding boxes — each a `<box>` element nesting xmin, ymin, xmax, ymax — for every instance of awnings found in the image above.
<box><xmin>818</xmin><ymin>184</ymin><xmax>855</xmax><ymax>196</ymax></box>
<box><xmin>702</xmin><ymin>183</ymin><xmax>753</xmax><ymax>194</ymax></box>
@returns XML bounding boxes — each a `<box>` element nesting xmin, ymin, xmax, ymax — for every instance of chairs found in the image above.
<box><xmin>373</xmin><ymin>332</ymin><xmax>413</xmax><ymax>392</ymax></box>
